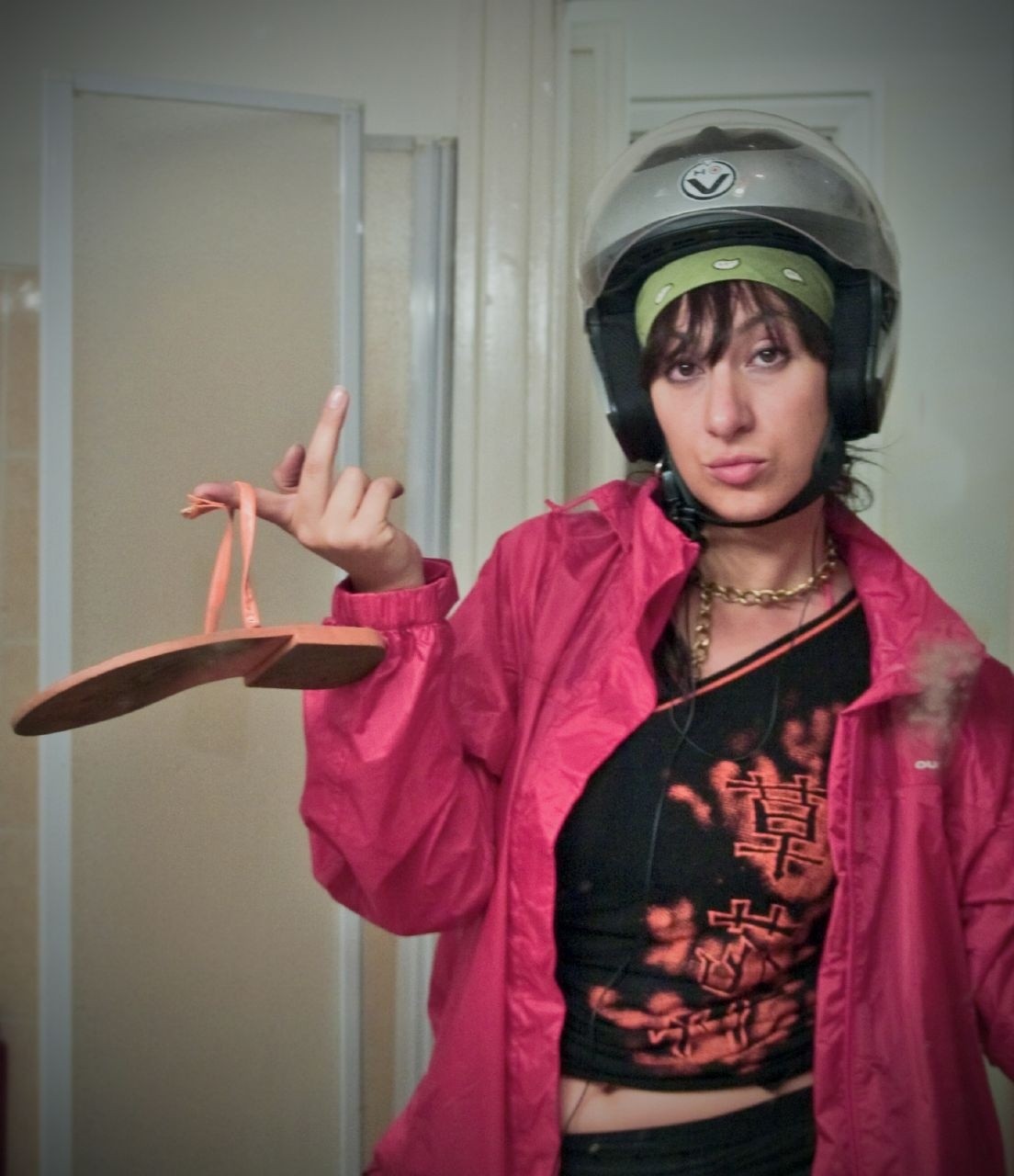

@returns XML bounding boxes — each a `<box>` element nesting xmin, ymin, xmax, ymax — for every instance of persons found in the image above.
<box><xmin>194</xmin><ymin>110</ymin><xmax>1013</xmax><ymax>1174</ymax></box>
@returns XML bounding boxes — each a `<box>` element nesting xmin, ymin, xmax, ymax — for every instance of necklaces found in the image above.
<box><xmin>690</xmin><ymin>537</ymin><xmax>839</xmax><ymax>682</ymax></box>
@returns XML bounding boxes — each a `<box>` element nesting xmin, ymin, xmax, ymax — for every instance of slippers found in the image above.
<box><xmin>11</xmin><ymin>480</ymin><xmax>386</xmax><ymax>737</ymax></box>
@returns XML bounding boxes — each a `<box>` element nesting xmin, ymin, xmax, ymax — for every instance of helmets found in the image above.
<box><xmin>578</xmin><ymin>108</ymin><xmax>900</xmax><ymax>465</ymax></box>
